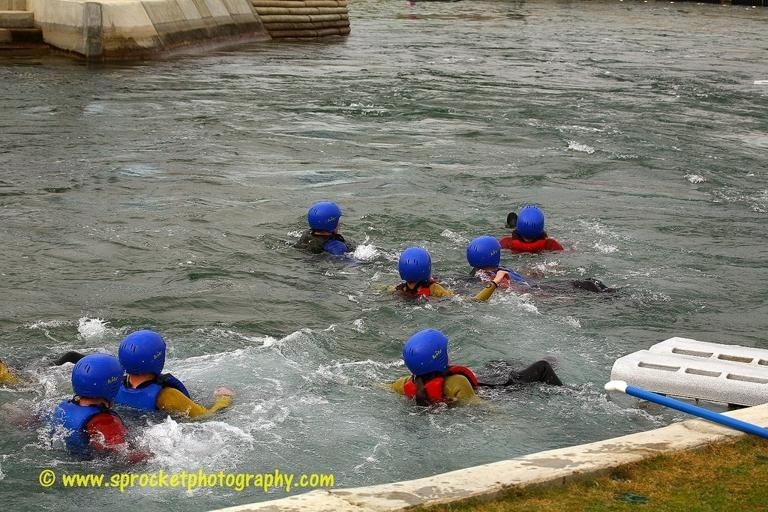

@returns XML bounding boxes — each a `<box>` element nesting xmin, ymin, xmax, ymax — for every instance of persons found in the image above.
<box><xmin>43</xmin><ymin>351</ymin><xmax>149</xmax><ymax>463</ymax></box>
<box><xmin>291</xmin><ymin>200</ymin><xmax>351</xmax><ymax>255</ymax></box>
<box><xmin>386</xmin><ymin>326</ymin><xmax>562</xmax><ymax>408</ymax></box>
<box><xmin>498</xmin><ymin>204</ymin><xmax>566</xmax><ymax>254</ymax></box>
<box><xmin>465</xmin><ymin>235</ymin><xmax>620</xmax><ymax>294</ymax></box>
<box><xmin>110</xmin><ymin>329</ymin><xmax>235</xmax><ymax>419</ymax></box>
<box><xmin>389</xmin><ymin>246</ymin><xmax>511</xmax><ymax>302</ymax></box>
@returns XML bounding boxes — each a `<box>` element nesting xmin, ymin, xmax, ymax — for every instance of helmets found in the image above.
<box><xmin>308</xmin><ymin>202</ymin><xmax>341</xmax><ymax>233</ymax></box>
<box><xmin>403</xmin><ymin>330</ymin><xmax>447</xmax><ymax>376</ymax></box>
<box><xmin>72</xmin><ymin>353</ymin><xmax>123</xmax><ymax>403</ymax></box>
<box><xmin>517</xmin><ymin>206</ymin><xmax>544</xmax><ymax>236</ymax></box>
<box><xmin>399</xmin><ymin>248</ymin><xmax>431</xmax><ymax>283</ymax></box>
<box><xmin>467</xmin><ymin>237</ymin><xmax>500</xmax><ymax>268</ymax></box>
<box><xmin>118</xmin><ymin>330</ymin><xmax>165</xmax><ymax>373</ymax></box>
